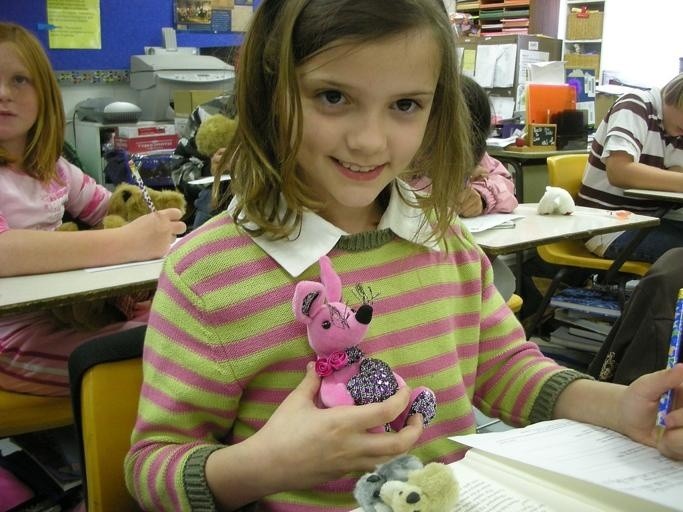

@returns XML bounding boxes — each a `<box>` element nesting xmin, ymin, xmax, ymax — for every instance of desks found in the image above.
<box><xmin>1</xmin><ymin>189</ymin><xmax>683</xmax><ymax>320</ymax></box>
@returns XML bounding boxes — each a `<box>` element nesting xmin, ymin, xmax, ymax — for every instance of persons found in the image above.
<box><xmin>0</xmin><ymin>20</ymin><xmax>186</xmax><ymax>398</ymax></box>
<box><xmin>403</xmin><ymin>75</ymin><xmax>519</xmax><ymax>219</ymax></box>
<box><xmin>123</xmin><ymin>0</ymin><xmax>683</xmax><ymax>512</ymax></box>
<box><xmin>169</xmin><ymin>95</ymin><xmax>238</xmax><ymax>230</ymax></box>
<box><xmin>576</xmin><ymin>72</ymin><xmax>683</xmax><ymax>265</ymax></box>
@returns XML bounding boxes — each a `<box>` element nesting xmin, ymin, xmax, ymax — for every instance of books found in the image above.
<box><xmin>345</xmin><ymin>419</ymin><xmax>682</xmax><ymax>512</ymax></box>
<box><xmin>7</xmin><ymin>436</ymin><xmax>82</xmax><ymax>492</ymax></box>
<box><xmin>454</xmin><ymin>0</ymin><xmax>530</xmax><ymax>35</ymax></box>
<box><xmin>524</xmin><ymin>286</ymin><xmax>624</xmax><ymax>371</ymax></box>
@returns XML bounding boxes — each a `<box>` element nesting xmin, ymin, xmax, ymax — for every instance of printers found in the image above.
<box><xmin>130</xmin><ymin>55</ymin><xmax>236</xmax><ymax>121</ymax></box>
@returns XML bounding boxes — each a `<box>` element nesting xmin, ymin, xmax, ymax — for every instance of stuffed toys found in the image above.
<box><xmin>290</xmin><ymin>255</ymin><xmax>436</xmax><ymax>433</ymax></box>
<box><xmin>535</xmin><ymin>184</ymin><xmax>576</xmax><ymax>216</ymax></box>
<box><xmin>43</xmin><ymin>179</ymin><xmax>185</xmax><ymax>333</ymax></box>
<box><xmin>193</xmin><ymin>114</ymin><xmax>238</xmax><ymax>158</ymax></box>
<box><xmin>354</xmin><ymin>452</ymin><xmax>458</xmax><ymax>512</ymax></box>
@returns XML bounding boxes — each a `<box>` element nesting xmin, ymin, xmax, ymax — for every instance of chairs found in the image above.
<box><xmin>524</xmin><ymin>154</ymin><xmax>658</xmax><ymax>342</ymax></box>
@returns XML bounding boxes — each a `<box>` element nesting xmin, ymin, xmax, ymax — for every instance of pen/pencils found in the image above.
<box><xmin>128</xmin><ymin>161</ymin><xmax>156</xmax><ymax>212</ymax></box>
<box><xmin>655</xmin><ymin>288</ymin><xmax>683</xmax><ymax>452</ymax></box>
